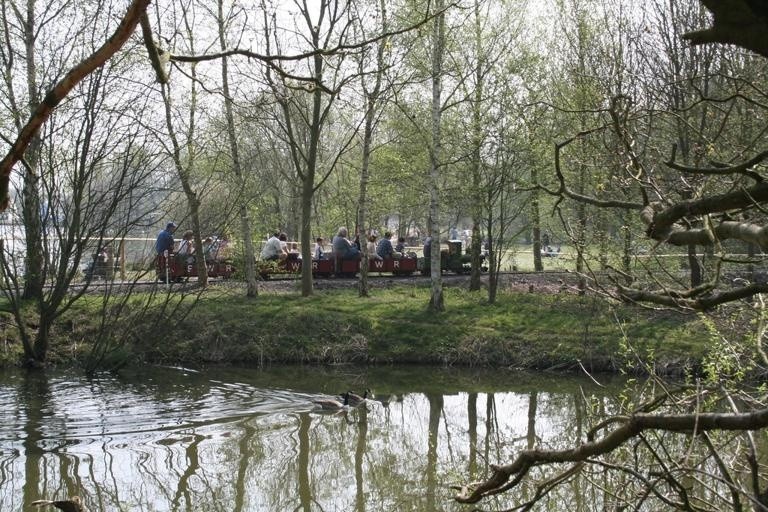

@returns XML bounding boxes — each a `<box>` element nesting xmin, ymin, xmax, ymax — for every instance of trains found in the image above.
<box><xmin>154</xmin><ymin>238</ymin><xmax>491</xmax><ymax>281</ymax></box>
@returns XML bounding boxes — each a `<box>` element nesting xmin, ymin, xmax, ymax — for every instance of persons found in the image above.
<box><xmin>259</xmin><ymin>226</ymin><xmax>473</xmax><ymax>262</ymax></box>
<box><xmin>542</xmin><ymin>228</ymin><xmax>561</xmax><ymax>257</ymax></box>
<box><xmin>155</xmin><ymin>222</ymin><xmax>225</xmax><ymax>263</ymax></box>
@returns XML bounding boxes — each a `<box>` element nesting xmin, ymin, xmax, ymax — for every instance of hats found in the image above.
<box><xmin>167</xmin><ymin>222</ymin><xmax>177</xmax><ymax>228</ymax></box>
